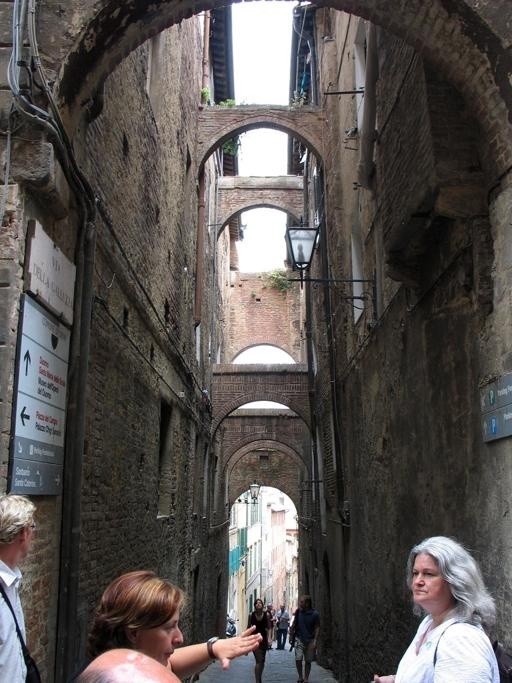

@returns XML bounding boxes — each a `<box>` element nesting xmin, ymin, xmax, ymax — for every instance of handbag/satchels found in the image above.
<box><xmin>24</xmin><ymin>652</ymin><xmax>42</xmax><ymax>683</ymax></box>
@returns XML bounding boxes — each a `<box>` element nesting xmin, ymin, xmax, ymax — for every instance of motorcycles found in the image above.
<box><xmin>226</xmin><ymin>613</ymin><xmax>240</xmax><ymax>636</ymax></box>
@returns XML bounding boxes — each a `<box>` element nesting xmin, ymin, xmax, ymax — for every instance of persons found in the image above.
<box><xmin>289</xmin><ymin>595</ymin><xmax>320</xmax><ymax>683</ymax></box>
<box><xmin>373</xmin><ymin>536</ymin><xmax>501</xmax><ymax>683</ymax></box>
<box><xmin>0</xmin><ymin>494</ymin><xmax>43</xmax><ymax>683</ymax></box>
<box><xmin>245</xmin><ymin>598</ymin><xmax>269</xmax><ymax>683</ymax></box>
<box><xmin>70</xmin><ymin>571</ymin><xmax>263</xmax><ymax>683</ymax></box>
<box><xmin>265</xmin><ymin>603</ymin><xmax>291</xmax><ymax>650</ymax></box>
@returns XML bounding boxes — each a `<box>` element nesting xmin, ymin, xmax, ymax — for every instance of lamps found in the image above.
<box><xmin>284</xmin><ymin>214</ymin><xmax>377</xmax><ymax>292</ymax></box>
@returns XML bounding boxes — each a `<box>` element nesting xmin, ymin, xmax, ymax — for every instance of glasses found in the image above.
<box><xmin>30</xmin><ymin>522</ymin><xmax>37</xmax><ymax>530</ymax></box>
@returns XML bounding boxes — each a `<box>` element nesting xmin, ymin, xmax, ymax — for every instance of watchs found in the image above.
<box><xmin>207</xmin><ymin>637</ymin><xmax>220</xmax><ymax>659</ymax></box>
